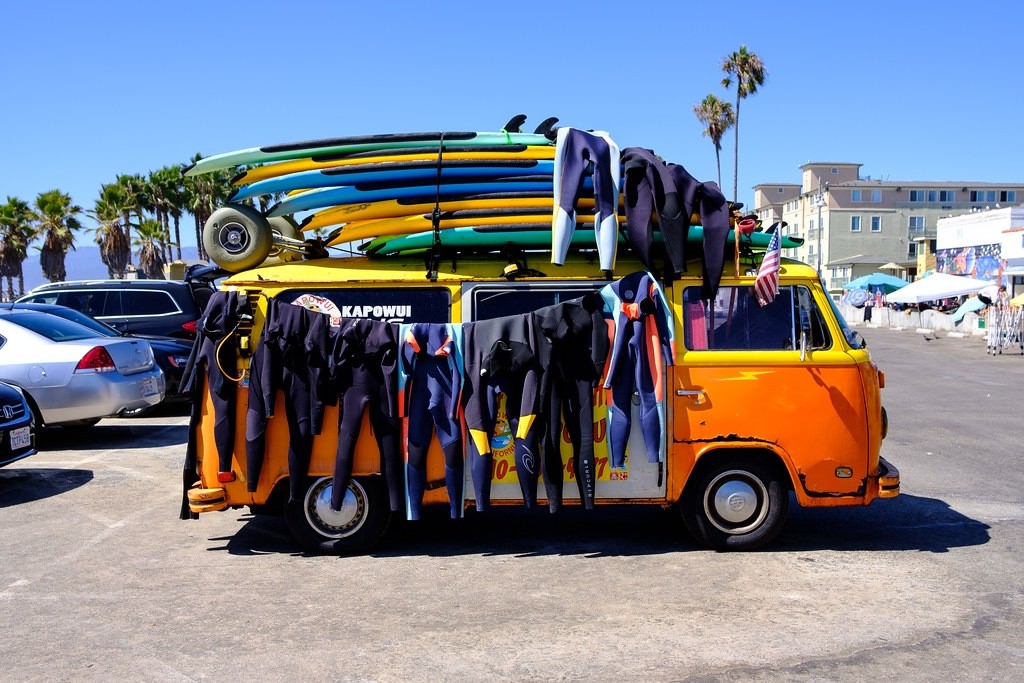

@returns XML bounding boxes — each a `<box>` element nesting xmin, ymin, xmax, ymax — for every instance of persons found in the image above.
<box><xmin>978</xmin><ymin>285</ymin><xmax>1006</xmax><ymax>305</ymax></box>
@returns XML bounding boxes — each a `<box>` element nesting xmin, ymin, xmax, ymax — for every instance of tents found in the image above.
<box><xmin>842</xmin><ymin>273</ymin><xmax>909</xmax><ymax>292</ymax></box>
<box><xmin>885</xmin><ymin>274</ymin><xmax>995</xmax><ymax>330</ymax></box>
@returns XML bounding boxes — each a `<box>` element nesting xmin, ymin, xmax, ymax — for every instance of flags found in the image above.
<box><xmin>754</xmin><ymin>225</ymin><xmax>780</xmax><ymax>308</ymax></box>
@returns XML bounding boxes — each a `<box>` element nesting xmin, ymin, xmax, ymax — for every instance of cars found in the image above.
<box><xmin>1</xmin><ymin>303</ymin><xmax>196</xmax><ymax>469</ymax></box>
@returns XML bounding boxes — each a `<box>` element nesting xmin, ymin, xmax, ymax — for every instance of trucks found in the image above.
<box><xmin>186</xmin><ymin>255</ymin><xmax>899</xmax><ymax>553</ymax></box>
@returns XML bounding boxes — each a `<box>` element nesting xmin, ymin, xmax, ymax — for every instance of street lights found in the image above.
<box><xmin>817</xmin><ymin>176</ymin><xmax>826</xmax><ymax>275</ymax></box>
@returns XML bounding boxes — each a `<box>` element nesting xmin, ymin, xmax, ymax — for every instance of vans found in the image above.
<box><xmin>12</xmin><ymin>279</ymin><xmax>215</xmax><ymax>339</ymax></box>
